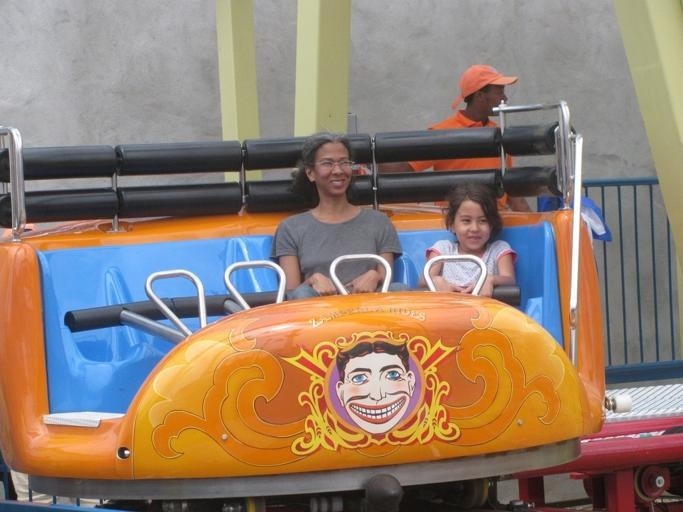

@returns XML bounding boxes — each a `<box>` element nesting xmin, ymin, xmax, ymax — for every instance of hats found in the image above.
<box><xmin>459</xmin><ymin>64</ymin><xmax>519</xmax><ymax>97</ymax></box>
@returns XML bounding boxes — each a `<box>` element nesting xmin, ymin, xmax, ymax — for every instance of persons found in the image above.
<box><xmin>398</xmin><ymin>63</ymin><xmax>532</xmax><ymax>213</ymax></box>
<box><xmin>270</xmin><ymin>130</ymin><xmax>403</xmax><ymax>303</ymax></box>
<box><xmin>417</xmin><ymin>179</ymin><xmax>518</xmax><ymax>308</ymax></box>
